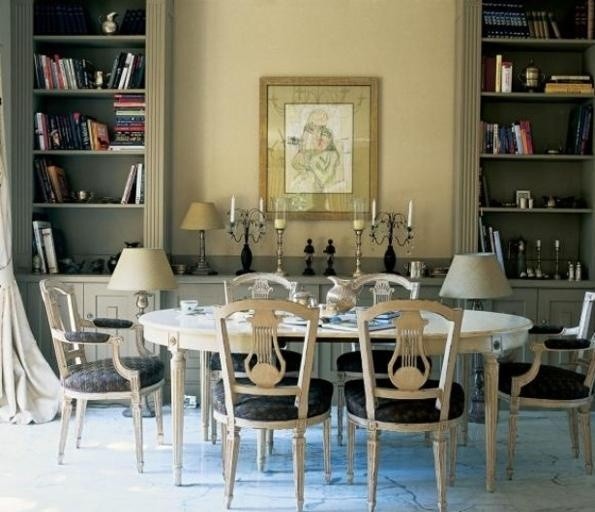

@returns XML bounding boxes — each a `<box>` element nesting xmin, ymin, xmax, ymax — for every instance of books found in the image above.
<box><xmin>33</xmin><ymin>109</ymin><xmax>109</xmax><ymax>151</ymax></box>
<box><xmin>34</xmin><ymin>156</ymin><xmax>71</xmax><ymax>203</ymax></box>
<box><xmin>542</xmin><ymin>73</ymin><xmax>595</xmax><ymax>95</ymax></box>
<box><xmin>118</xmin><ymin>9</ymin><xmax>146</xmax><ymax>36</ymax></box>
<box><xmin>572</xmin><ymin>0</ymin><xmax>595</xmax><ymax>39</ymax></box>
<box><xmin>480</xmin><ymin>117</ymin><xmax>535</xmax><ymax>154</ymax></box>
<box><xmin>481</xmin><ymin>0</ymin><xmax>561</xmax><ymax>40</ymax></box>
<box><xmin>33</xmin><ymin>0</ymin><xmax>100</xmax><ymax>36</ymax></box>
<box><xmin>108</xmin><ymin>92</ymin><xmax>145</xmax><ymax>153</ymax></box>
<box><xmin>120</xmin><ymin>160</ymin><xmax>145</xmax><ymax>206</ymax></box>
<box><xmin>481</xmin><ymin>174</ymin><xmax>491</xmax><ymax>208</ymax></box>
<box><xmin>105</xmin><ymin>50</ymin><xmax>145</xmax><ymax>90</ymax></box>
<box><xmin>478</xmin><ymin>215</ymin><xmax>507</xmax><ymax>278</ymax></box>
<box><xmin>31</xmin><ymin>218</ymin><xmax>58</xmax><ymax>276</ymax></box>
<box><xmin>565</xmin><ymin>103</ymin><xmax>594</xmax><ymax>155</ymax></box>
<box><xmin>480</xmin><ymin>52</ymin><xmax>514</xmax><ymax>93</ymax></box>
<box><xmin>33</xmin><ymin>50</ymin><xmax>96</xmax><ymax>90</ymax></box>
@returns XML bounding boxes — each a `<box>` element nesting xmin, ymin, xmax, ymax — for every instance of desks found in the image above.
<box><xmin>132</xmin><ymin>302</ymin><xmax>534</xmax><ymax>494</ymax></box>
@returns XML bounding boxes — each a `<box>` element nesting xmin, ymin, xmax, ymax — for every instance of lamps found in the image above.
<box><xmin>177</xmin><ymin>199</ymin><xmax>228</xmax><ymax>277</ymax></box>
<box><xmin>438</xmin><ymin>249</ymin><xmax>515</xmax><ymax>425</ymax></box>
<box><xmin>105</xmin><ymin>245</ymin><xmax>178</xmax><ymax>420</ymax></box>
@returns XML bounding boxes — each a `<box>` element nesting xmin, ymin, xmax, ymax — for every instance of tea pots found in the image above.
<box><xmin>325</xmin><ymin>275</ymin><xmax>359</xmax><ymax>313</ymax></box>
<box><xmin>293</xmin><ymin>285</ymin><xmax>316</xmax><ymax>322</ymax></box>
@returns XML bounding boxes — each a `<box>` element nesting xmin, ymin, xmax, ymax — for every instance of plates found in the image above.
<box><xmin>321</xmin><ymin>321</ymin><xmax>397</xmax><ymax>332</ymax></box>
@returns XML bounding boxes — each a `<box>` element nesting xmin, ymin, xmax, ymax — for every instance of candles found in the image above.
<box><xmin>351</xmin><ymin>197</ymin><xmax>367</xmax><ymax>232</ymax></box>
<box><xmin>406</xmin><ymin>199</ymin><xmax>414</xmax><ymax>231</ymax></box>
<box><xmin>534</xmin><ymin>239</ymin><xmax>543</xmax><ymax>252</ymax></box>
<box><xmin>273</xmin><ymin>201</ymin><xmax>288</xmax><ymax>230</ymax></box>
<box><xmin>553</xmin><ymin>238</ymin><xmax>560</xmax><ymax>252</ymax></box>
<box><xmin>228</xmin><ymin>195</ymin><xmax>237</xmax><ymax>224</ymax></box>
<box><xmin>370</xmin><ymin>198</ymin><xmax>379</xmax><ymax>230</ymax></box>
<box><xmin>257</xmin><ymin>198</ymin><xmax>266</xmax><ymax>227</ymax></box>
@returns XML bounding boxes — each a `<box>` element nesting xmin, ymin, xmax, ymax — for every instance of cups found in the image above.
<box><xmin>411</xmin><ymin>261</ymin><xmax>425</xmax><ymax>279</ymax></box>
<box><xmin>180</xmin><ymin>300</ymin><xmax>197</xmax><ymax>313</ymax></box>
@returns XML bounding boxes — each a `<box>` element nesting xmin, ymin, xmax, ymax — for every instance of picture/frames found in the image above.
<box><xmin>257</xmin><ymin>76</ymin><xmax>381</xmax><ymax>226</ymax></box>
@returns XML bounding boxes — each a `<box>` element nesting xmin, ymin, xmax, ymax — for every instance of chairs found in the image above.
<box><xmin>206</xmin><ymin>296</ymin><xmax>333</xmax><ymax>512</ymax></box>
<box><xmin>486</xmin><ymin>292</ymin><xmax>595</xmax><ymax>484</ymax></box>
<box><xmin>341</xmin><ymin>299</ymin><xmax>464</xmax><ymax>510</ymax></box>
<box><xmin>36</xmin><ymin>276</ymin><xmax>166</xmax><ymax>477</ymax></box>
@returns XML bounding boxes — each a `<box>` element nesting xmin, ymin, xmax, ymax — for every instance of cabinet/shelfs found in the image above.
<box><xmin>7</xmin><ymin>0</ymin><xmax>169</xmax><ymax>379</ymax></box>
<box><xmin>451</xmin><ymin>1</ymin><xmax>595</xmax><ymax>389</ymax></box>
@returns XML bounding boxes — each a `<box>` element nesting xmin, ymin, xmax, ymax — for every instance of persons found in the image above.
<box><xmin>290</xmin><ymin>106</ymin><xmax>345</xmax><ymax>192</ymax></box>
